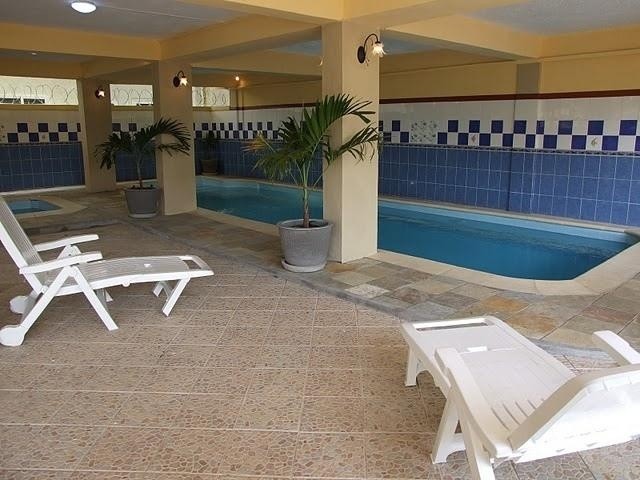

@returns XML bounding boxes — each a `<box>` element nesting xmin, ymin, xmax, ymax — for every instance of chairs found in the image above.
<box><xmin>1</xmin><ymin>196</ymin><xmax>214</xmax><ymax>346</ymax></box>
<box><xmin>399</xmin><ymin>315</ymin><xmax>639</xmax><ymax>479</ymax></box>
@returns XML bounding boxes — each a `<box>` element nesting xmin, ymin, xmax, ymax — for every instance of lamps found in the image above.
<box><xmin>95</xmin><ymin>87</ymin><xmax>105</xmax><ymax>99</ymax></box>
<box><xmin>173</xmin><ymin>71</ymin><xmax>188</xmax><ymax>88</ymax></box>
<box><xmin>357</xmin><ymin>33</ymin><xmax>387</xmax><ymax>64</ymax></box>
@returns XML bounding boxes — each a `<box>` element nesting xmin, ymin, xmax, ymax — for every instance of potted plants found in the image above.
<box><xmin>198</xmin><ymin>133</ymin><xmax>219</xmax><ymax>176</ymax></box>
<box><xmin>93</xmin><ymin>117</ymin><xmax>192</xmax><ymax>218</ymax></box>
<box><xmin>243</xmin><ymin>93</ymin><xmax>386</xmax><ymax>272</ymax></box>
